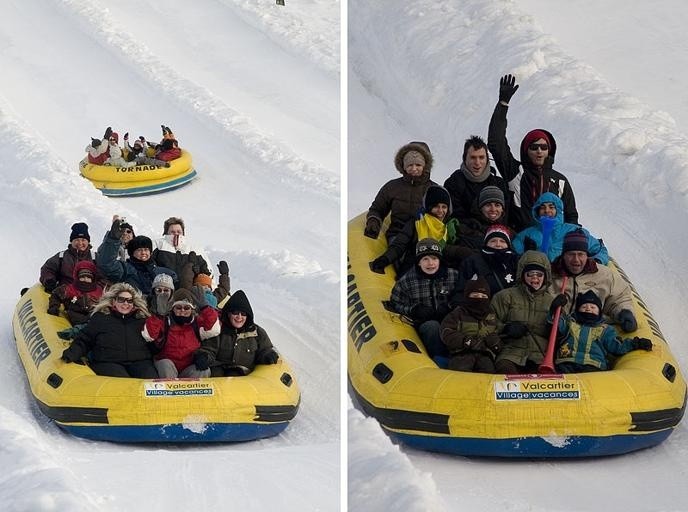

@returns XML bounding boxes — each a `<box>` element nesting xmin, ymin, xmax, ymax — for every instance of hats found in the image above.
<box><xmin>152</xmin><ymin>273</ymin><xmax>219</xmax><ymax>310</ymax></box>
<box><xmin>128</xmin><ymin>236</ymin><xmax>152</xmax><ymax>255</ymax></box>
<box><xmin>146</xmin><ymin>148</ymin><xmax>156</xmax><ymax>157</ymax></box>
<box><xmin>121</xmin><ymin>223</ymin><xmax>134</xmax><ymax>237</ymax></box>
<box><xmin>70</xmin><ymin>223</ymin><xmax>90</xmax><ymax>242</ymax></box>
<box><xmin>91</xmin><ymin>137</ymin><xmax>101</xmax><ymax>147</ymax></box>
<box><xmin>78</xmin><ymin>269</ymin><xmax>92</xmax><ymax>277</ymax></box>
<box><xmin>403</xmin><ymin>130</ymin><xmax>601</xmax><ymax>309</ymax></box>
<box><xmin>134</xmin><ymin>140</ymin><xmax>143</xmax><ymax>147</ymax></box>
<box><xmin>112</xmin><ymin>133</ymin><xmax>118</xmax><ymax>144</ymax></box>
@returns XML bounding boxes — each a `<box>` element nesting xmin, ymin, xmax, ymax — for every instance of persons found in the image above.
<box><xmin>512</xmin><ymin>192</ymin><xmax>610</xmax><ymax>268</ymax></box>
<box><xmin>364</xmin><ymin>129</ymin><xmax>562</xmax><ymax>375</ymax></box>
<box><xmin>486</xmin><ymin>75</ymin><xmax>584</xmax><ymax>229</ymax></box>
<box><xmin>85</xmin><ymin>123</ymin><xmax>184</xmax><ymax>171</ymax></box>
<box><xmin>541</xmin><ymin>231</ymin><xmax>636</xmax><ymax>332</ymax></box>
<box><xmin>40</xmin><ymin>213</ymin><xmax>280</xmax><ymax>380</ymax></box>
<box><xmin>546</xmin><ymin>290</ymin><xmax>652</xmax><ymax>372</ymax></box>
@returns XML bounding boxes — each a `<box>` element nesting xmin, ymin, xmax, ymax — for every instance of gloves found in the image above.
<box><xmin>104</xmin><ymin>127</ymin><xmax>112</xmax><ymax>140</ymax></box>
<box><xmin>505</xmin><ymin>321</ymin><xmax>525</xmax><ymax>338</ymax></box>
<box><xmin>217</xmin><ymin>261</ymin><xmax>228</xmax><ymax>274</ymax></box>
<box><xmin>124</xmin><ymin>133</ymin><xmax>128</xmax><ymax>140</ymax></box>
<box><xmin>500</xmin><ymin>75</ymin><xmax>518</xmax><ymax>103</ymax></box>
<box><xmin>415</xmin><ymin>305</ymin><xmax>449</xmax><ymax>320</ymax></box>
<box><xmin>465</xmin><ymin>333</ymin><xmax>500</xmax><ymax>352</ymax></box>
<box><xmin>619</xmin><ymin>309</ymin><xmax>652</xmax><ymax>350</ymax></box>
<box><xmin>47</xmin><ymin>306</ymin><xmax>60</xmax><ymax>314</ymax></box>
<box><xmin>373</xmin><ymin>255</ymin><xmax>389</xmax><ymax>272</ymax></box>
<box><xmin>364</xmin><ymin>218</ymin><xmax>380</xmax><ymax>238</ymax></box>
<box><xmin>139</xmin><ymin>136</ymin><xmax>144</xmax><ymax>141</ymax></box>
<box><xmin>110</xmin><ymin>219</ymin><xmax>124</xmax><ymax>240</ymax></box>
<box><xmin>262</xmin><ymin>351</ymin><xmax>278</xmax><ymax>364</ymax></box>
<box><xmin>161</xmin><ymin>125</ymin><xmax>172</xmax><ymax>135</ymax></box>
<box><xmin>194</xmin><ymin>353</ymin><xmax>209</xmax><ymax>371</ymax></box>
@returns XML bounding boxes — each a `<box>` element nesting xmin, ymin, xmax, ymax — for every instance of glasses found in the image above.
<box><xmin>118</xmin><ymin>296</ymin><xmax>134</xmax><ymax>303</ymax></box>
<box><xmin>232</xmin><ymin>310</ymin><xmax>246</xmax><ymax>315</ymax></box>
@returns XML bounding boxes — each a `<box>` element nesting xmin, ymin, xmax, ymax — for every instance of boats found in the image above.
<box><xmin>10</xmin><ymin>277</ymin><xmax>308</xmax><ymax>450</ymax></box>
<box><xmin>347</xmin><ymin>206</ymin><xmax>688</xmax><ymax>467</ymax></box>
<box><xmin>75</xmin><ymin>142</ymin><xmax>199</xmax><ymax>199</ymax></box>
